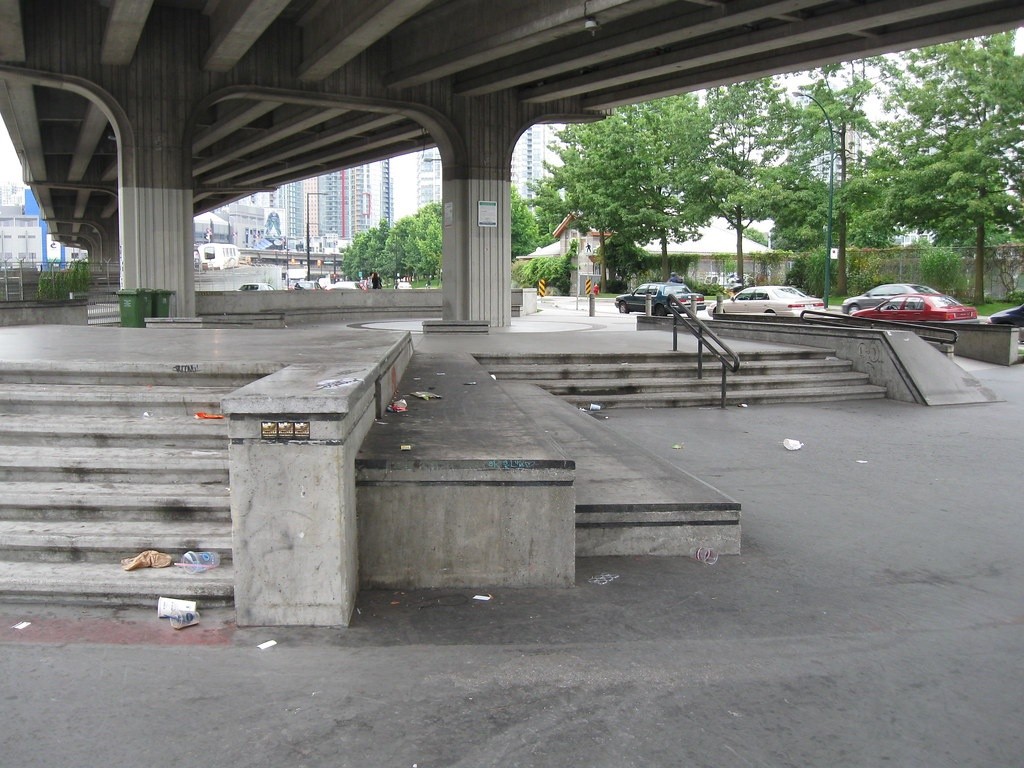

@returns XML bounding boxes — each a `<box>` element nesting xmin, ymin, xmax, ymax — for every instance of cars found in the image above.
<box><xmin>396</xmin><ymin>282</ymin><xmax>412</xmax><ymax>289</ymax></box>
<box><xmin>613</xmin><ymin>282</ymin><xmax>707</xmax><ymax>317</ymax></box>
<box><xmin>704</xmin><ymin>286</ymin><xmax>826</xmax><ymax>317</ymax></box>
<box><xmin>293</xmin><ymin>279</ymin><xmax>368</xmax><ymax>289</ymax></box>
<box><xmin>841</xmin><ymin>284</ymin><xmax>941</xmax><ymax>316</ymax></box>
<box><xmin>985</xmin><ymin>303</ymin><xmax>1024</xmax><ymax>330</ymax></box>
<box><xmin>850</xmin><ymin>293</ymin><xmax>977</xmax><ymax>322</ymax></box>
<box><xmin>236</xmin><ymin>283</ymin><xmax>276</xmax><ymax>291</ymax></box>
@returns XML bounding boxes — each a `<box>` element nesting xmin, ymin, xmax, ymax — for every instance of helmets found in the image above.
<box><xmin>670</xmin><ymin>272</ymin><xmax>676</xmax><ymax>275</ymax></box>
<box><xmin>727</xmin><ymin>273</ymin><xmax>734</xmax><ymax>276</ymax></box>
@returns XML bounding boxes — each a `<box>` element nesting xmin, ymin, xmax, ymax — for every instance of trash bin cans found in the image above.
<box><xmin>116</xmin><ymin>288</ymin><xmax>155</xmax><ymax>328</ymax></box>
<box><xmin>152</xmin><ymin>289</ymin><xmax>176</xmax><ymax>317</ymax></box>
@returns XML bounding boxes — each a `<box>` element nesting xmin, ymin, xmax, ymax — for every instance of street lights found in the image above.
<box><xmin>383</xmin><ymin>229</ymin><xmax>399</xmax><ymax>289</ymax></box>
<box><xmin>306</xmin><ymin>192</ymin><xmax>330</xmax><ymax>281</ymax></box>
<box><xmin>790</xmin><ymin>91</ymin><xmax>835</xmax><ymax>309</ymax></box>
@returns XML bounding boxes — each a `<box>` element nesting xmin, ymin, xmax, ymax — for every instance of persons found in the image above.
<box><xmin>366</xmin><ymin>272</ymin><xmax>382</xmax><ymax>289</ymax></box>
<box><xmin>594</xmin><ymin>283</ymin><xmax>600</xmax><ymax>295</ymax></box>
<box><xmin>668</xmin><ymin>272</ymin><xmax>683</xmax><ymax>283</ymax></box>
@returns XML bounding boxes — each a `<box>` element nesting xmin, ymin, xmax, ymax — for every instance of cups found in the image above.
<box><xmin>590</xmin><ymin>404</ymin><xmax>601</xmax><ymax>411</ymax></box>
<box><xmin>157</xmin><ymin>596</ymin><xmax>200</xmax><ymax>629</ymax></box>
<box><xmin>784</xmin><ymin>439</ymin><xmax>801</xmax><ymax>450</ymax></box>
<box><xmin>182</xmin><ymin>551</ymin><xmax>221</xmax><ymax>575</ymax></box>
